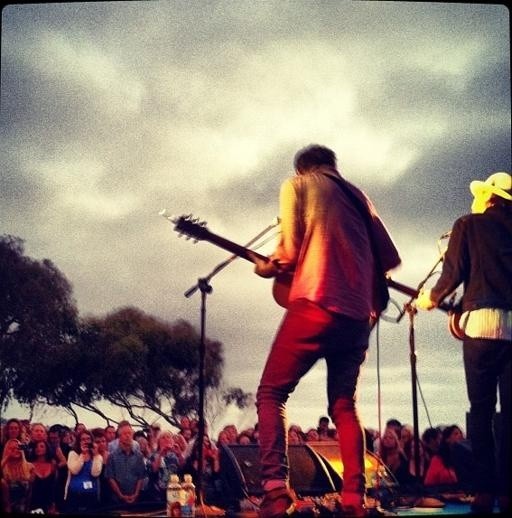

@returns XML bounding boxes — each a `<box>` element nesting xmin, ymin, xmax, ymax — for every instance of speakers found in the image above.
<box><xmin>218</xmin><ymin>440</ymin><xmax>337</xmax><ymax>512</ymax></box>
<box><xmin>306</xmin><ymin>441</ymin><xmax>401</xmax><ymax>509</ymax></box>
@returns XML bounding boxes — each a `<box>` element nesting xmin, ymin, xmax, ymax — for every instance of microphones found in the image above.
<box><xmin>438</xmin><ymin>230</ymin><xmax>452</xmax><ymax>241</ymax></box>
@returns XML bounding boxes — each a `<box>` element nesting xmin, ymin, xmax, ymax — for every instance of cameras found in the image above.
<box><xmin>143</xmin><ymin>428</ymin><xmax>150</xmax><ymax>432</ymax></box>
<box><xmin>19</xmin><ymin>444</ymin><xmax>28</xmax><ymax>450</ymax></box>
<box><xmin>88</xmin><ymin>442</ymin><xmax>94</xmax><ymax>449</ymax></box>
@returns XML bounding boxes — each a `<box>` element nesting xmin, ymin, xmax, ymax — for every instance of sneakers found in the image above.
<box><xmin>471</xmin><ymin>494</ymin><xmax>510</xmax><ymax>513</ymax></box>
<box><xmin>343</xmin><ymin>505</ymin><xmax>367</xmax><ymax>517</ymax></box>
<box><xmin>263</xmin><ymin>498</ymin><xmax>287</xmax><ymax>517</ymax></box>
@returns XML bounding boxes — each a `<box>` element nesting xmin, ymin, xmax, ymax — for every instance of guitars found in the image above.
<box><xmin>384</xmin><ymin>277</ymin><xmax>463</xmax><ymax>340</ymax></box>
<box><xmin>170</xmin><ymin>215</ymin><xmax>291</xmax><ymax>308</ymax></box>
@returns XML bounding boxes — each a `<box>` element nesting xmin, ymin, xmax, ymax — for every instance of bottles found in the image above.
<box><xmin>181</xmin><ymin>472</ymin><xmax>197</xmax><ymax>518</ymax></box>
<box><xmin>164</xmin><ymin>472</ymin><xmax>181</xmax><ymax>517</ymax></box>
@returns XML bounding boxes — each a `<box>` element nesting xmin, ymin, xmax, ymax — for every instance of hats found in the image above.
<box><xmin>470</xmin><ymin>172</ymin><xmax>512</xmax><ymax>201</ymax></box>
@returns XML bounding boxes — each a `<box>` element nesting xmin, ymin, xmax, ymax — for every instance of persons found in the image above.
<box><xmin>249</xmin><ymin>143</ymin><xmax>381</xmax><ymax>518</ymax></box>
<box><xmin>289</xmin><ymin>417</ymin><xmax>337</xmax><ymax>443</ymax></box>
<box><xmin>365</xmin><ymin>419</ymin><xmax>464</xmax><ymax>500</ymax></box>
<box><xmin>0</xmin><ymin>415</ymin><xmax>219</xmax><ymax>518</ymax></box>
<box><xmin>216</xmin><ymin>425</ymin><xmax>261</xmax><ymax>447</ymax></box>
<box><xmin>411</xmin><ymin>171</ymin><xmax>512</xmax><ymax>518</ymax></box>
